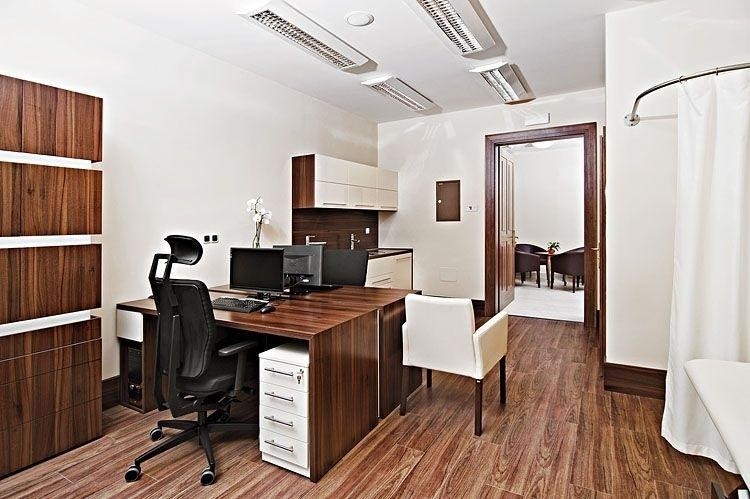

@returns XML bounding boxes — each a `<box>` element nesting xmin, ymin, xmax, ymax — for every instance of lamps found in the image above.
<box><xmin>236</xmin><ymin>0</ymin><xmax>532</xmax><ymax>111</ymax></box>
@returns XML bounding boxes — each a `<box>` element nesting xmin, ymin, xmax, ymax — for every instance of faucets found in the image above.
<box><xmin>305</xmin><ymin>235</ymin><xmax>328</xmax><ymax>246</ymax></box>
<box><xmin>350</xmin><ymin>232</ymin><xmax>361</xmax><ymax>250</ymax></box>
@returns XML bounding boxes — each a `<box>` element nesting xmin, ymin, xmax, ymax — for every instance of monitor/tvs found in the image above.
<box><xmin>229</xmin><ymin>247</ymin><xmax>284</xmax><ymax>303</ymax></box>
<box><xmin>272</xmin><ymin>245</ymin><xmax>324</xmax><ymax>296</ymax></box>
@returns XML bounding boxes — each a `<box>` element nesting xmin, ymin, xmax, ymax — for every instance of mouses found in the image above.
<box><xmin>260</xmin><ymin>306</ymin><xmax>276</xmax><ymax>313</ymax></box>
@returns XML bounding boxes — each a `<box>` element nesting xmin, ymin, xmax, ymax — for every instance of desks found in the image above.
<box><xmin>534</xmin><ymin>251</ymin><xmax>556</xmax><ymax>287</ymax></box>
<box><xmin>207</xmin><ymin>276</ymin><xmax>424</xmax><ymax>420</ymax></box>
<box><xmin>114</xmin><ymin>288</ymin><xmax>377</xmax><ymax>483</ymax></box>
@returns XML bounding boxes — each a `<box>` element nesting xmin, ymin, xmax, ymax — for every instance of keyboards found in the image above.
<box><xmin>298</xmin><ymin>285</ymin><xmax>343</xmax><ymax>291</ymax></box>
<box><xmin>210</xmin><ymin>297</ymin><xmax>268</xmax><ymax>314</ymax></box>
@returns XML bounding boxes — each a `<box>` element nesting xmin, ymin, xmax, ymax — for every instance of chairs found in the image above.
<box><xmin>126</xmin><ymin>232</ymin><xmax>260</xmax><ymax>487</ymax></box>
<box><xmin>515</xmin><ymin>249</ymin><xmax>541</xmax><ymax>287</ymax></box>
<box><xmin>516</xmin><ymin>242</ymin><xmax>547</xmax><ymax>281</ymax></box>
<box><xmin>550</xmin><ymin>246</ymin><xmax>584</xmax><ymax>293</ymax></box>
<box><xmin>322</xmin><ymin>250</ymin><xmax>370</xmax><ymax>286</ymax></box>
<box><xmin>398</xmin><ymin>293</ymin><xmax>510</xmax><ymax>435</ymax></box>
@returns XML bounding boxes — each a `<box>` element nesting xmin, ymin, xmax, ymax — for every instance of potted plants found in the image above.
<box><xmin>547</xmin><ymin>241</ymin><xmax>560</xmax><ymax>254</ymax></box>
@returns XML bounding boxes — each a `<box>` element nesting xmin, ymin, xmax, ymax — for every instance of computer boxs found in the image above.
<box><xmin>125</xmin><ymin>346</ymin><xmax>142</xmax><ymax>408</ymax></box>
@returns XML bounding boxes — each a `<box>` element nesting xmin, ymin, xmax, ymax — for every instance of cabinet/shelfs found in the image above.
<box><xmin>684</xmin><ymin>358</ymin><xmax>750</xmax><ymax>499</ymax></box>
<box><xmin>0</xmin><ymin>69</ymin><xmax>104</xmax><ymax>478</ymax></box>
<box><xmin>292</xmin><ymin>154</ymin><xmax>398</xmax><ymax>211</ymax></box>
<box><xmin>258</xmin><ymin>340</ymin><xmax>310</xmax><ymax>480</ymax></box>
<box><xmin>365</xmin><ymin>246</ymin><xmax>413</xmax><ymax>288</ymax></box>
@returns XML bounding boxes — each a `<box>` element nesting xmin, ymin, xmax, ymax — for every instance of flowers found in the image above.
<box><xmin>246</xmin><ymin>191</ymin><xmax>272</xmax><ymax>248</ymax></box>
